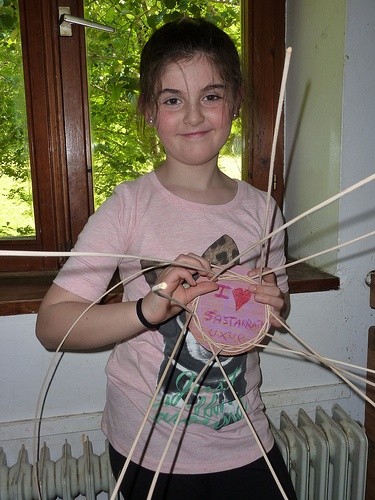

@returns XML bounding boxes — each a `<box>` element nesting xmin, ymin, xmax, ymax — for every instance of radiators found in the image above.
<box><xmin>1</xmin><ymin>403</ymin><xmax>369</xmax><ymax>500</ymax></box>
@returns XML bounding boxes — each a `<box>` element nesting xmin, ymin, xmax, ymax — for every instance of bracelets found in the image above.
<box><xmin>136</xmin><ymin>298</ymin><xmax>161</xmax><ymax>330</ymax></box>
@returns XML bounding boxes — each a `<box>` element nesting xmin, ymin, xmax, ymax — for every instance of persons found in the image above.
<box><xmin>35</xmin><ymin>18</ymin><xmax>300</xmax><ymax>500</ymax></box>
